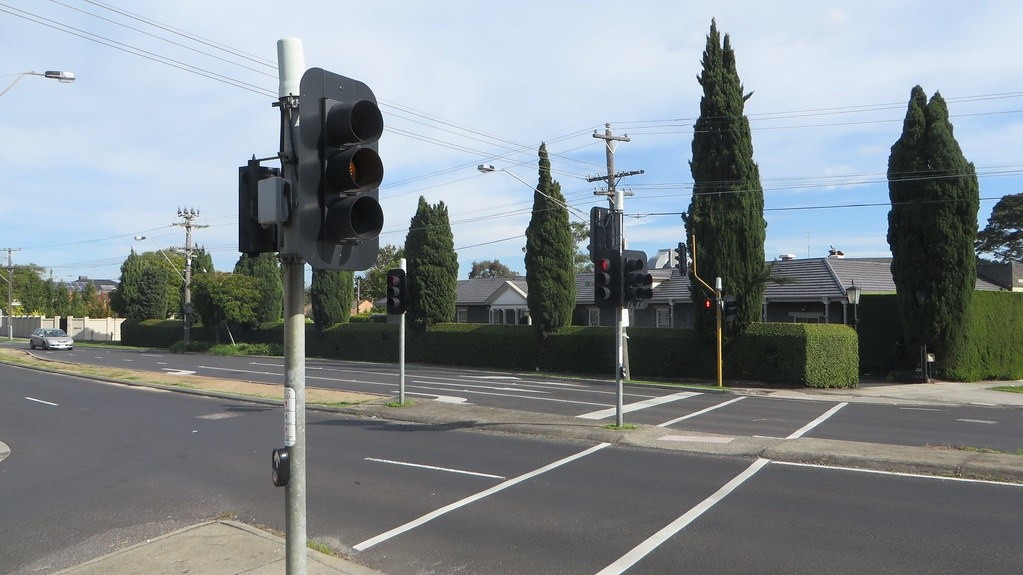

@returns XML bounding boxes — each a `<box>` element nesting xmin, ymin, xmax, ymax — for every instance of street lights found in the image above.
<box><xmin>477</xmin><ymin>162</ymin><xmax>632</xmax><ymax>381</ymax></box>
<box><xmin>134</xmin><ymin>236</ymin><xmax>190</xmax><ymax>347</ymax></box>
<box><xmin>846</xmin><ymin>276</ymin><xmax>861</xmax><ymax>333</ymax></box>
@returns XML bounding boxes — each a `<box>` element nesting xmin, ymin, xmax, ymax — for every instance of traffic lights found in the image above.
<box><xmin>703</xmin><ymin>298</ymin><xmax>714</xmax><ymax>311</ymax></box>
<box><xmin>386</xmin><ymin>268</ymin><xmax>407</xmax><ymax>315</ymax></box>
<box><xmin>586</xmin><ymin>206</ymin><xmax>609</xmax><ymax>265</ymax></box>
<box><xmin>622</xmin><ymin>249</ymin><xmax>653</xmax><ymax>310</ymax></box>
<box><xmin>294</xmin><ymin>67</ymin><xmax>385</xmax><ymax>271</ymax></box>
<box><xmin>675</xmin><ymin>242</ymin><xmax>687</xmax><ymax>276</ymax></box>
<box><xmin>594</xmin><ymin>250</ymin><xmax>622</xmax><ymax>309</ymax></box>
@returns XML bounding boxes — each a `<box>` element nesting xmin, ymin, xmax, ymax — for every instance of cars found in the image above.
<box><xmin>29</xmin><ymin>328</ymin><xmax>74</xmax><ymax>350</ymax></box>
